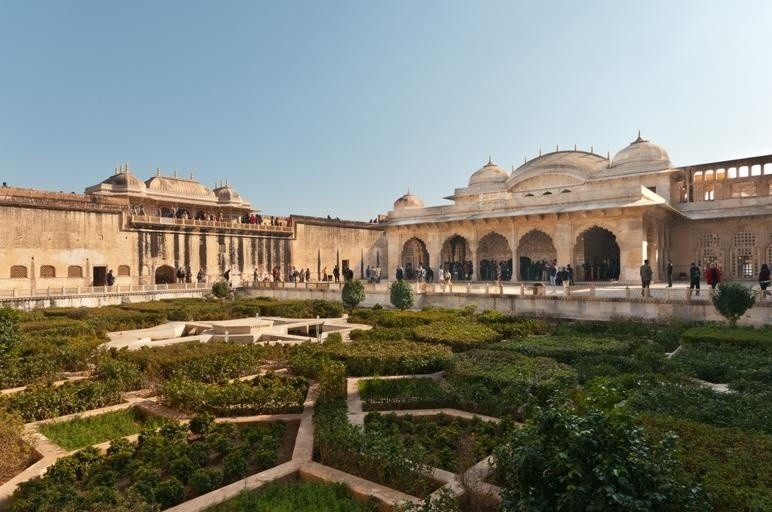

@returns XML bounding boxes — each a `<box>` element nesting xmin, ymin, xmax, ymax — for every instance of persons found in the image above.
<box><xmin>690</xmin><ymin>262</ymin><xmax>721</xmax><ymax>295</ymax></box>
<box><xmin>229</xmin><ymin>283</ymin><xmax>234</xmax><ymax>301</ymax></box>
<box><xmin>328</xmin><ymin>215</ymin><xmax>341</xmax><ymax>220</ymax></box>
<box><xmin>2</xmin><ymin>182</ymin><xmax>8</xmax><ymax>187</ymax></box>
<box><xmin>759</xmin><ymin>264</ymin><xmax>772</xmax><ymax>298</ymax></box>
<box><xmin>370</xmin><ymin>218</ymin><xmax>388</xmax><ymax>223</ymax></box>
<box><xmin>667</xmin><ymin>260</ymin><xmax>673</xmax><ymax>287</ymax></box>
<box><xmin>177</xmin><ymin>265</ymin><xmax>381</xmax><ymax>284</ymax></box>
<box><xmin>107</xmin><ymin>269</ymin><xmax>116</xmax><ymax>286</ymax></box>
<box><xmin>640</xmin><ymin>260</ymin><xmax>653</xmax><ymax>297</ymax></box>
<box><xmin>396</xmin><ymin>258</ymin><xmax>620</xmax><ymax>288</ymax></box>
<box><xmin>131</xmin><ymin>204</ymin><xmax>292</xmax><ymax>227</ymax></box>
